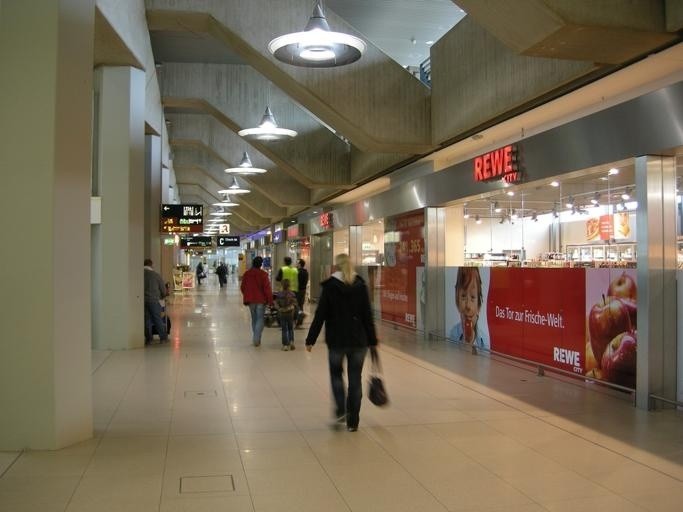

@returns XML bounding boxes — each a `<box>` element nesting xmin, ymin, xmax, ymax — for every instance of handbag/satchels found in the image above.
<box><xmin>370</xmin><ymin>377</ymin><xmax>388</xmax><ymax>406</ymax></box>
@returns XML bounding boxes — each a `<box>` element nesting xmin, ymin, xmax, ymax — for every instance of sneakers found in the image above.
<box><xmin>282</xmin><ymin>345</ymin><xmax>288</xmax><ymax>350</ymax></box>
<box><xmin>290</xmin><ymin>342</ymin><xmax>295</xmax><ymax>350</ymax></box>
<box><xmin>348</xmin><ymin>425</ymin><xmax>358</xmax><ymax>432</ymax></box>
<box><xmin>337</xmin><ymin>410</ymin><xmax>347</xmax><ymax>420</ymax></box>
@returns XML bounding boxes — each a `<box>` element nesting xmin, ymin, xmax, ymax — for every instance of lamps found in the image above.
<box><xmin>462</xmin><ymin>185</ymin><xmax>634</xmax><ymax>226</ymax></box>
<box><xmin>265</xmin><ymin>1</ymin><xmax>369</xmax><ymax>69</ymax></box>
<box><xmin>208</xmin><ymin>83</ymin><xmax>299</xmax><ymax>216</ymax></box>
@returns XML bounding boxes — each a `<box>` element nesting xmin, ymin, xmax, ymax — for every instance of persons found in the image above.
<box><xmin>294</xmin><ymin>259</ymin><xmax>309</xmax><ymax>310</ymax></box>
<box><xmin>272</xmin><ymin>278</ymin><xmax>300</xmax><ymax>351</ymax></box>
<box><xmin>143</xmin><ymin>258</ymin><xmax>169</xmax><ymax>345</ymax></box>
<box><xmin>239</xmin><ymin>255</ymin><xmax>274</xmax><ymax>350</ymax></box>
<box><xmin>274</xmin><ymin>256</ymin><xmax>306</xmax><ymax>324</ymax></box>
<box><xmin>304</xmin><ymin>253</ymin><xmax>378</xmax><ymax>432</ymax></box>
<box><xmin>448</xmin><ymin>267</ymin><xmax>488</xmax><ymax>353</ymax></box>
<box><xmin>196</xmin><ymin>260</ymin><xmax>228</xmax><ymax>287</ymax></box>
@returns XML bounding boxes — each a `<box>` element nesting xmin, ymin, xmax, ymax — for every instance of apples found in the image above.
<box><xmin>586</xmin><ymin>270</ymin><xmax>637</xmax><ymax>394</ymax></box>
<box><xmin>465</xmin><ymin>320</ymin><xmax>473</xmax><ymax>337</ymax></box>
